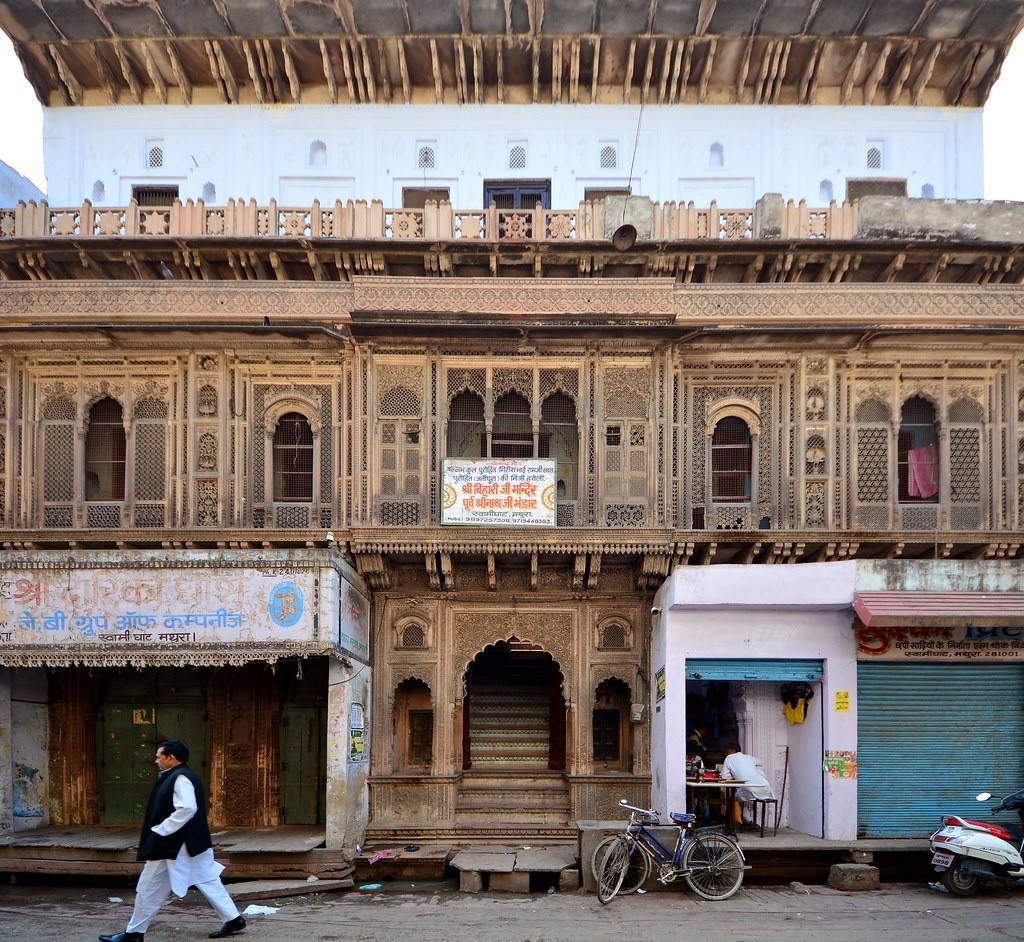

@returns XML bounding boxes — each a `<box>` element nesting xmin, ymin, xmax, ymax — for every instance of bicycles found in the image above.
<box><xmin>591</xmin><ymin>799</ymin><xmax>752</xmax><ymax>904</ymax></box>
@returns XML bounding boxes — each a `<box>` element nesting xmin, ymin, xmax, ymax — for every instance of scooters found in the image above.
<box><xmin>929</xmin><ymin>788</ymin><xmax>1024</xmax><ymax>896</ymax></box>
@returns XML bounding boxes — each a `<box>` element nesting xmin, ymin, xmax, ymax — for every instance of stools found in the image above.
<box><xmin>743</xmin><ymin>799</ymin><xmax>779</xmax><ymax>838</ymax></box>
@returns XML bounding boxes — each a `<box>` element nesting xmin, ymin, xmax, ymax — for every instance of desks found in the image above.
<box><xmin>687</xmin><ymin>782</ymin><xmax>766</xmax><ymax>840</ymax></box>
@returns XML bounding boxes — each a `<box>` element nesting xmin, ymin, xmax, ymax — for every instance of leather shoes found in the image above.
<box><xmin>208</xmin><ymin>919</ymin><xmax>246</xmax><ymax>939</ymax></box>
<box><xmin>99</xmin><ymin>928</ymin><xmax>146</xmax><ymax>942</ymax></box>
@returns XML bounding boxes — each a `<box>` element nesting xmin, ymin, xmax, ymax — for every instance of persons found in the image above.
<box><xmin>720</xmin><ymin>742</ymin><xmax>775</xmax><ymax>827</ymax></box>
<box><xmin>99</xmin><ymin>737</ymin><xmax>247</xmax><ymax>942</ymax></box>
<box><xmin>686</xmin><ymin>735</ymin><xmax>713</xmax><ymax>824</ymax></box>
